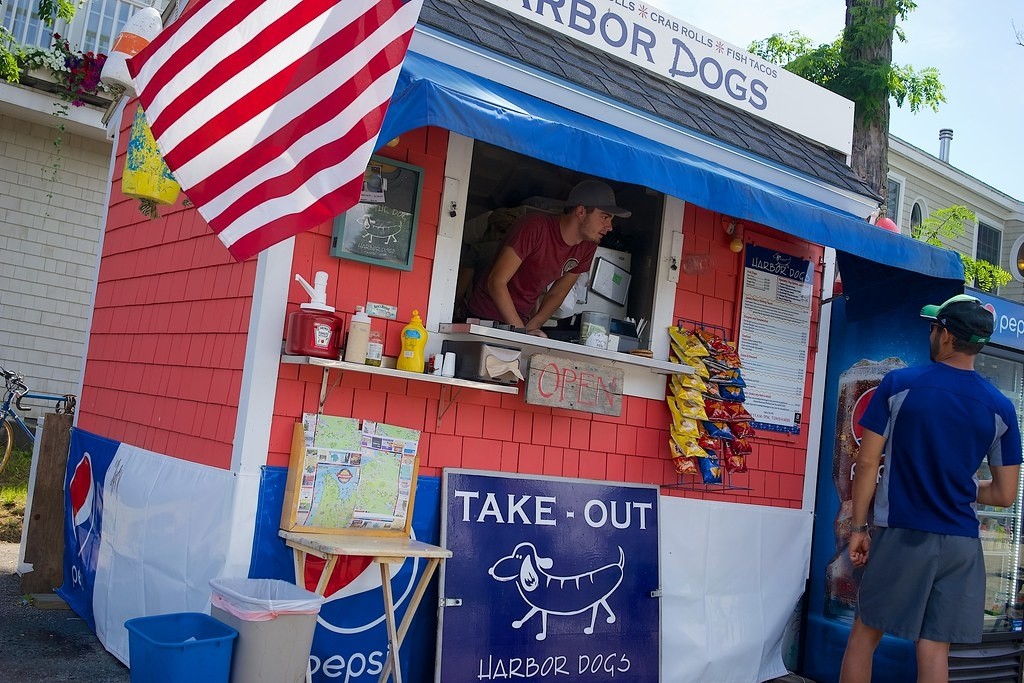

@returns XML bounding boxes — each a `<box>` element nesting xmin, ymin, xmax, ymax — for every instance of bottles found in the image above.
<box><xmin>344</xmin><ymin>306</ymin><xmax>371</xmax><ymax>364</ymax></box>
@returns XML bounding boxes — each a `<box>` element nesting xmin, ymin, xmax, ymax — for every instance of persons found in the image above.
<box><xmin>470</xmin><ymin>178</ymin><xmax>631</xmax><ymax>337</ymax></box>
<box><xmin>454</xmin><ymin>208</ymin><xmax>521</xmax><ymax>322</ymax></box>
<box><xmin>839</xmin><ymin>293</ymin><xmax>1023</xmax><ymax>683</ymax></box>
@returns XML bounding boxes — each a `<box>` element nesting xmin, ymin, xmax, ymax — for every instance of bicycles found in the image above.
<box><xmin>0</xmin><ymin>367</ymin><xmax>76</xmax><ymax>474</ymax></box>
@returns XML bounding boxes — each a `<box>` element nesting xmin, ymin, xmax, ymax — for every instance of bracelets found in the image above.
<box><xmin>852</xmin><ymin>523</ymin><xmax>868</xmax><ymax>531</ymax></box>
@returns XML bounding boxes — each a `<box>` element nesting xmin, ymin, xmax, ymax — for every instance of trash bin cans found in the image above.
<box><xmin>210</xmin><ymin>577</ymin><xmax>327</xmax><ymax>683</ymax></box>
<box><xmin>123</xmin><ymin>611</ymin><xmax>240</xmax><ymax>683</ymax></box>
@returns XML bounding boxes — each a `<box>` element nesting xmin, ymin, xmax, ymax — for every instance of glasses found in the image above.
<box><xmin>930</xmin><ymin>322</ymin><xmax>949</xmax><ymax>334</ymax></box>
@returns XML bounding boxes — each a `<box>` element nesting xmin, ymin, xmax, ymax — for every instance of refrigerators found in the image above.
<box><xmin>797</xmin><ymin>281</ymin><xmax>1024</xmax><ymax>683</ymax></box>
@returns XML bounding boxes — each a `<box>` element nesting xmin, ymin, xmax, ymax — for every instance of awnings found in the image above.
<box><xmin>374</xmin><ymin>51</ymin><xmax>964</xmax><ymax>283</ymax></box>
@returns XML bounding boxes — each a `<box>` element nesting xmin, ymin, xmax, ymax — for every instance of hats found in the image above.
<box><xmin>569</xmin><ymin>180</ymin><xmax>631</xmax><ymax>218</ymax></box>
<box><xmin>484</xmin><ymin>208</ymin><xmax>521</xmax><ymax>232</ymax></box>
<box><xmin>920</xmin><ymin>294</ymin><xmax>994</xmax><ymax>344</ymax></box>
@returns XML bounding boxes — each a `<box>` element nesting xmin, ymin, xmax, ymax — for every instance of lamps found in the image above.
<box><xmin>720</xmin><ymin>212</ymin><xmax>743</xmax><ymax>251</ymax></box>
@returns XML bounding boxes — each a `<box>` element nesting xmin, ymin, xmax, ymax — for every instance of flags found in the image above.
<box><xmin>126</xmin><ymin>0</ymin><xmax>424</xmax><ymax>264</ymax></box>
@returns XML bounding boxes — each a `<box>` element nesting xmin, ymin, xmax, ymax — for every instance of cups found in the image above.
<box><xmin>826</xmin><ymin>365</ymin><xmax>901</xmax><ymax>624</ymax></box>
<box><xmin>480</xmin><ymin>320</ymin><xmax>499</xmax><ymax>329</ymax></box>
<box><xmin>515</xmin><ymin>328</ymin><xmax>527</xmax><ymax>334</ymax></box>
<box><xmin>498</xmin><ymin>325</ymin><xmax>516</xmax><ymax>332</ymax></box>
<box><xmin>442</xmin><ymin>352</ymin><xmax>456</xmax><ymax>376</ymax></box>
<box><xmin>581</xmin><ymin>312</ymin><xmax>610</xmax><ymax>347</ymax></box>
<box><xmin>467</xmin><ymin>318</ymin><xmax>480</xmax><ymax>325</ymax></box>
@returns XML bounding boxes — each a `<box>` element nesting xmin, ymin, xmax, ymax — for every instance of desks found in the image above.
<box><xmin>278</xmin><ymin>528</ymin><xmax>453</xmax><ymax>683</ymax></box>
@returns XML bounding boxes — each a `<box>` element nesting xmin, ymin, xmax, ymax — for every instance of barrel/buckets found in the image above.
<box><xmin>123</xmin><ymin>612</ymin><xmax>236</xmax><ymax>683</ymax></box>
